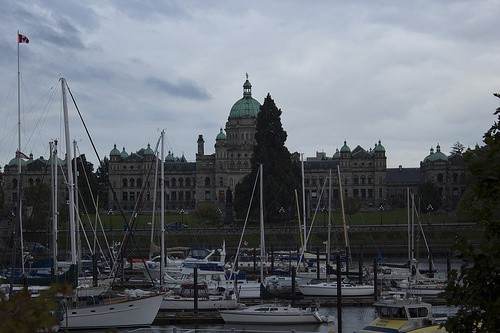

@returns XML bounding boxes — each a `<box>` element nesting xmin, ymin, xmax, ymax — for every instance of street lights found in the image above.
<box><xmin>427</xmin><ymin>204</ymin><xmax>433</xmax><ymax>225</ymax></box>
<box><xmin>321</xmin><ymin>206</ymin><xmax>328</xmax><ymax>227</ymax></box>
<box><xmin>278</xmin><ymin>207</ymin><xmax>285</xmax><ymax>228</ymax></box>
<box><xmin>108</xmin><ymin>209</ymin><xmax>114</xmax><ymax>230</ymax></box>
<box><xmin>216</xmin><ymin>208</ymin><xmax>222</xmax><ymax>229</ymax></box>
<box><xmin>378</xmin><ymin>204</ymin><xmax>384</xmax><ymax>225</ymax></box>
<box><xmin>180</xmin><ymin>209</ymin><xmax>186</xmax><ymax>224</ymax></box>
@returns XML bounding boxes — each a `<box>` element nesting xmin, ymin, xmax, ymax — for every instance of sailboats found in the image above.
<box><xmin>0</xmin><ymin>77</ymin><xmax>483</xmax><ymax>333</ymax></box>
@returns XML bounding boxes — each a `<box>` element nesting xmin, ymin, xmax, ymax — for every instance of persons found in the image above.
<box><xmin>295</xmin><ymin>284</ymin><xmax>301</xmax><ymax>302</ymax></box>
<box><xmin>58</xmin><ymin>269</ymin><xmax>62</xmax><ymax>275</ymax></box>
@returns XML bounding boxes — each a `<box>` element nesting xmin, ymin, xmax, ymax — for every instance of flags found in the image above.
<box><xmin>18</xmin><ymin>34</ymin><xmax>30</xmax><ymax>44</ymax></box>
